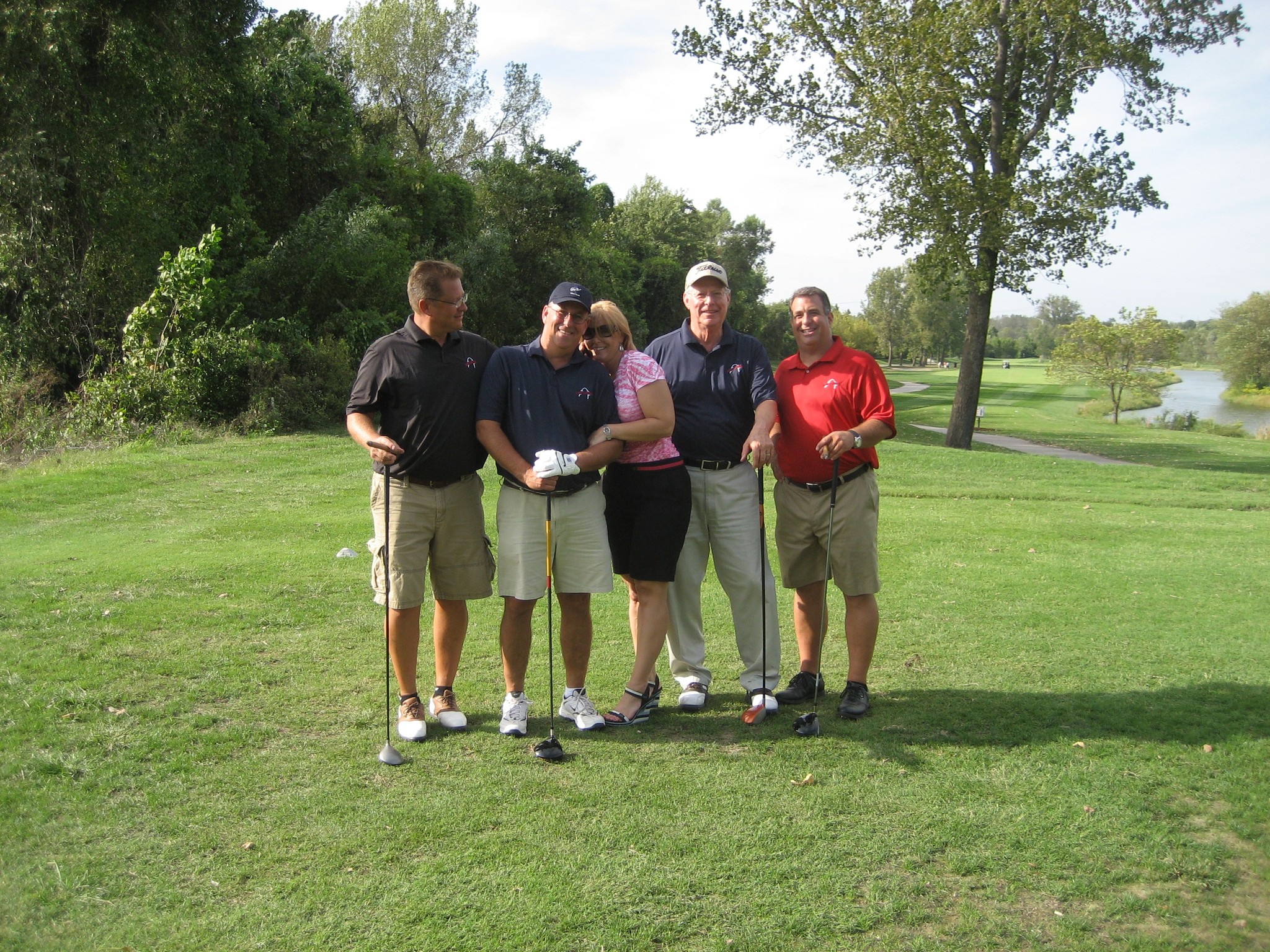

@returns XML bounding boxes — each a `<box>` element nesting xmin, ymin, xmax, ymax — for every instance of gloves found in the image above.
<box><xmin>532</xmin><ymin>447</ymin><xmax>580</xmax><ymax>478</ymax></box>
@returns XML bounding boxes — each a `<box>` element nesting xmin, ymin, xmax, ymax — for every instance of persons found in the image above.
<box><xmin>937</xmin><ymin>361</ymin><xmax>949</xmax><ymax>369</ymax></box>
<box><xmin>643</xmin><ymin>261</ymin><xmax>778</xmax><ymax>712</ymax></box>
<box><xmin>346</xmin><ymin>260</ymin><xmax>500</xmax><ymax>739</ymax></box>
<box><xmin>475</xmin><ymin>281</ymin><xmax>691</xmax><ymax>736</ymax></box>
<box><xmin>768</xmin><ymin>286</ymin><xmax>897</xmax><ymax>719</ymax></box>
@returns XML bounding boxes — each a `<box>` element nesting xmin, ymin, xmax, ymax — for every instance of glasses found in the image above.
<box><xmin>688</xmin><ymin>289</ymin><xmax>727</xmax><ymax>302</ymax></box>
<box><xmin>549</xmin><ymin>306</ymin><xmax>589</xmax><ymax>325</ymax></box>
<box><xmin>423</xmin><ymin>292</ymin><xmax>468</xmax><ymax>308</ymax></box>
<box><xmin>582</xmin><ymin>324</ymin><xmax>621</xmax><ymax>339</ymax></box>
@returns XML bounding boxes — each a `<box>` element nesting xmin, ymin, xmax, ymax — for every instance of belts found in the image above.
<box><xmin>396</xmin><ymin>477</ymin><xmax>460</xmax><ymax>490</ymax></box>
<box><xmin>683</xmin><ymin>458</ymin><xmax>745</xmax><ymax>471</ymax></box>
<box><xmin>606</xmin><ymin>459</ymin><xmax>683</xmax><ymax>471</ymax></box>
<box><xmin>786</xmin><ymin>464</ymin><xmax>868</xmax><ymax>493</ymax></box>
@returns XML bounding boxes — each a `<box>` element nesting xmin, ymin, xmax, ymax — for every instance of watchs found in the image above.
<box><xmin>847</xmin><ymin>430</ymin><xmax>862</xmax><ymax>448</ymax></box>
<box><xmin>603</xmin><ymin>424</ymin><xmax>611</xmax><ymax>440</ymax></box>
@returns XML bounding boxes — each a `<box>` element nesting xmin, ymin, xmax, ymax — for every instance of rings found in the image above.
<box><xmin>766</xmin><ymin>454</ymin><xmax>770</xmax><ymax>456</ymax></box>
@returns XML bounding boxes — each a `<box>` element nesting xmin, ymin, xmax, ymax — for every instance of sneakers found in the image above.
<box><xmin>743</xmin><ymin>687</ymin><xmax>778</xmax><ymax>711</ymax></box>
<box><xmin>499</xmin><ymin>691</ymin><xmax>534</xmax><ymax>736</ymax></box>
<box><xmin>678</xmin><ymin>682</ymin><xmax>708</xmax><ymax>708</ymax></box>
<box><xmin>429</xmin><ymin>689</ymin><xmax>466</xmax><ymax>728</ymax></box>
<box><xmin>397</xmin><ymin>697</ymin><xmax>426</xmax><ymax>740</ymax></box>
<box><xmin>558</xmin><ymin>684</ymin><xmax>606</xmax><ymax>730</ymax></box>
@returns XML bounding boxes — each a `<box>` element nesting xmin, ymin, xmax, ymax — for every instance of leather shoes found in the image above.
<box><xmin>775</xmin><ymin>672</ymin><xmax>825</xmax><ymax>703</ymax></box>
<box><xmin>836</xmin><ymin>681</ymin><xmax>869</xmax><ymax>716</ymax></box>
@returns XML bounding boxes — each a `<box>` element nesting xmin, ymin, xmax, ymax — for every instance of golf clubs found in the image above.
<box><xmin>378</xmin><ymin>464</ymin><xmax>405</xmax><ymax>765</ymax></box>
<box><xmin>791</xmin><ymin>456</ymin><xmax>842</xmax><ymax>735</ymax></box>
<box><xmin>532</xmin><ymin>491</ymin><xmax>565</xmax><ymax>760</ymax></box>
<box><xmin>740</xmin><ymin>465</ymin><xmax>766</xmax><ymax>725</ymax></box>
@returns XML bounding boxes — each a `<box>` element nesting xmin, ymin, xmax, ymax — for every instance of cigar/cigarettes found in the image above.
<box><xmin>822</xmin><ymin>436</ymin><xmax>829</xmax><ymax>459</ymax></box>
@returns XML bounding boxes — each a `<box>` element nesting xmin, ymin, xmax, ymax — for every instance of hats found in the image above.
<box><xmin>549</xmin><ymin>282</ymin><xmax>592</xmax><ymax>314</ymax></box>
<box><xmin>685</xmin><ymin>261</ymin><xmax>728</xmax><ymax>290</ymax></box>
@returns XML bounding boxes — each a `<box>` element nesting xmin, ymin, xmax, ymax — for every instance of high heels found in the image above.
<box><xmin>603</xmin><ymin>686</ymin><xmax>654</xmax><ymax>725</ymax></box>
<box><xmin>647</xmin><ymin>674</ymin><xmax>662</xmax><ymax>709</ymax></box>
